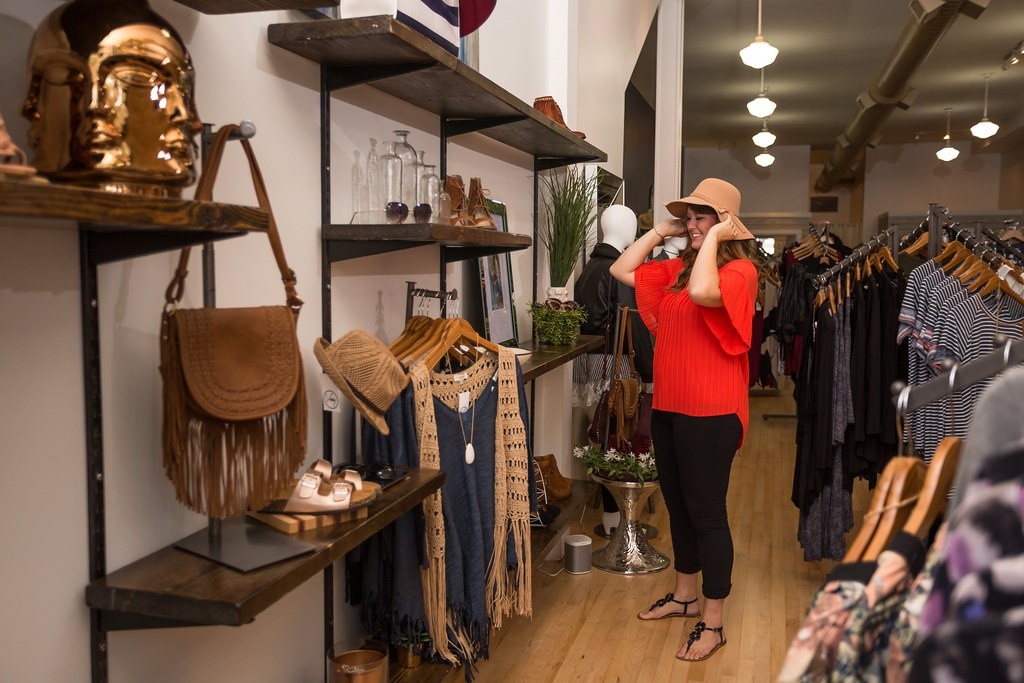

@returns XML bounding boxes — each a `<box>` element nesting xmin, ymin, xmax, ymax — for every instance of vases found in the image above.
<box><xmin>584</xmin><ymin>474</ymin><xmax>670</xmax><ymax>574</ymax></box>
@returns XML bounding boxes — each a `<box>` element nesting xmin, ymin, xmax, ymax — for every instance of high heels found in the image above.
<box><xmin>530</xmin><ymin>453</ymin><xmax>574</xmax><ymax>501</ymax></box>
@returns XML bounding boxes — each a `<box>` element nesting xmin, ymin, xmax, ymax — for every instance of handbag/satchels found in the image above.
<box><xmin>588</xmin><ymin>305</ymin><xmax>652</xmax><ymax>457</ymax></box>
<box><xmin>158</xmin><ymin>124</ymin><xmax>311</xmax><ymax>524</ymax></box>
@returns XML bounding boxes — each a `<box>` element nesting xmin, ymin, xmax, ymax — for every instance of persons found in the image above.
<box><xmin>22</xmin><ymin>0</ymin><xmax>198</xmax><ymax>195</ymax></box>
<box><xmin>607</xmin><ymin>177</ymin><xmax>777</xmax><ymax>659</ymax></box>
<box><xmin>573</xmin><ymin>205</ymin><xmax>652</xmax><ymax>534</ymax></box>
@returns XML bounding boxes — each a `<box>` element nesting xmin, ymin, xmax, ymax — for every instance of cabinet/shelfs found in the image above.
<box><xmin>269</xmin><ymin>16</ymin><xmax>607</xmax><ymax>251</ymax></box>
<box><xmin>0</xmin><ymin>0</ymin><xmax>448</xmax><ymax>629</ymax></box>
<box><xmin>506</xmin><ymin>332</ymin><xmax>601</xmax><ymax>575</ymax></box>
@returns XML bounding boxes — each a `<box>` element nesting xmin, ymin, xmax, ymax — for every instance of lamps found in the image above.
<box><xmin>738</xmin><ymin>0</ymin><xmax>780</xmax><ymax>167</ymax></box>
<box><xmin>935</xmin><ymin>107</ymin><xmax>960</xmax><ymax>163</ymax></box>
<box><xmin>969</xmin><ymin>74</ymin><xmax>1000</xmax><ymax>138</ymax></box>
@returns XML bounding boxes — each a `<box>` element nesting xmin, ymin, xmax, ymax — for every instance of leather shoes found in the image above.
<box><xmin>529</xmin><ymin>503</ymin><xmax>561</xmax><ymax>525</ymax></box>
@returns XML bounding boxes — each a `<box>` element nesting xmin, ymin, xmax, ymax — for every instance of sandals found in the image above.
<box><xmin>465</xmin><ymin>176</ymin><xmax>498</xmax><ymax>231</ymax></box>
<box><xmin>439</xmin><ymin>174</ymin><xmax>478</xmax><ymax>231</ymax></box>
<box><xmin>637</xmin><ymin>591</ymin><xmax>701</xmax><ymax>620</ymax></box>
<box><xmin>675</xmin><ymin>619</ymin><xmax>728</xmax><ymax>662</ymax></box>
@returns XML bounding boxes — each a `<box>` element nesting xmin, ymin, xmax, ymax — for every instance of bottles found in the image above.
<box><xmin>374</xmin><ymin>129</ymin><xmax>451</xmax><ymax>226</ymax></box>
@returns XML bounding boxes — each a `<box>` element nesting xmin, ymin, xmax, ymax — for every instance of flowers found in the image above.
<box><xmin>573</xmin><ymin>445</ymin><xmax>660</xmax><ymax>485</ymax></box>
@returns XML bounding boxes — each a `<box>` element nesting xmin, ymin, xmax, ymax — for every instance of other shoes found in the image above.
<box><xmin>0</xmin><ymin>112</ymin><xmax>39</xmax><ymax>180</ymax></box>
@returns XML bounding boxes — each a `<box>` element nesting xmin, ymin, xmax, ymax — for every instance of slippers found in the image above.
<box><xmin>244</xmin><ymin>458</ymin><xmax>383</xmax><ymax>516</ymax></box>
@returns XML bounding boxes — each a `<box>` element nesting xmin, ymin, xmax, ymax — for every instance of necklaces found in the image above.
<box><xmin>443</xmin><ymin>333</ymin><xmax>480</xmax><ymax>463</ymax></box>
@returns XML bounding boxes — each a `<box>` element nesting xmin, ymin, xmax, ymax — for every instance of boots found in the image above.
<box><xmin>532</xmin><ymin>95</ymin><xmax>587</xmax><ymax>139</ymax></box>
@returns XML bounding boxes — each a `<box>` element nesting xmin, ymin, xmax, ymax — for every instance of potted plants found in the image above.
<box><xmin>533</xmin><ymin>160</ymin><xmax>605</xmax><ymax>345</ymax></box>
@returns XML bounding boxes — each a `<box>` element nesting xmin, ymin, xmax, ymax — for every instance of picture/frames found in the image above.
<box><xmin>477</xmin><ymin>198</ymin><xmax>519</xmax><ymax>349</ymax></box>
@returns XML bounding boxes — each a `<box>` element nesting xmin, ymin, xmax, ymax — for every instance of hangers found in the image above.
<box><xmin>786</xmin><ymin>208</ymin><xmax>1024</xmax><ymax>579</ymax></box>
<box><xmin>390</xmin><ymin>289</ymin><xmax>510</xmax><ymax>373</ymax></box>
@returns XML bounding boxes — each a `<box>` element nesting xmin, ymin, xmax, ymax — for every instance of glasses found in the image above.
<box><xmin>331</xmin><ymin>460</ymin><xmax>397</xmax><ymax>482</ymax></box>
<box><xmin>545</xmin><ymin>297</ymin><xmax>579</xmax><ymax>313</ymax></box>
<box><xmin>348</xmin><ymin>201</ymin><xmax>435</xmax><ymax>226</ymax></box>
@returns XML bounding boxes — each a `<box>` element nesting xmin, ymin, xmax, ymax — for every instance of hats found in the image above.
<box><xmin>660</xmin><ymin>177</ymin><xmax>756</xmax><ymax>248</ymax></box>
<box><xmin>313</xmin><ymin>328</ymin><xmax>408</xmax><ymax>436</ymax></box>
<box><xmin>637</xmin><ymin>213</ymin><xmax>654</xmax><ymax>230</ymax></box>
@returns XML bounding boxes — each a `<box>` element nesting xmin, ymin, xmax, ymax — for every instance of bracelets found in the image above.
<box><xmin>653</xmin><ymin>226</ymin><xmax>665</xmax><ymax>240</ymax></box>
